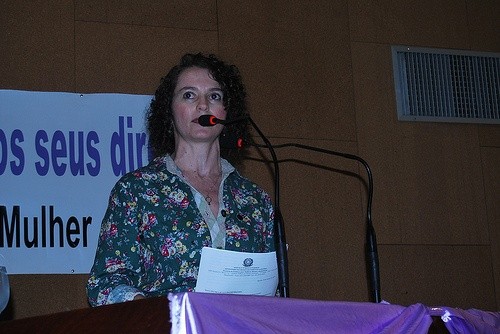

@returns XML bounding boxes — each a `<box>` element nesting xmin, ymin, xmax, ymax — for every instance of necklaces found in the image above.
<box><xmin>182</xmin><ymin>171</ymin><xmax>222</xmax><ymax>206</ymax></box>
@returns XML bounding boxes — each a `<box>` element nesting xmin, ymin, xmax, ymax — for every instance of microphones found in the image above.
<box><xmin>198</xmin><ymin>113</ymin><xmax>291</xmax><ymax>297</ymax></box>
<box><xmin>225</xmin><ymin>135</ymin><xmax>381</xmax><ymax>304</ymax></box>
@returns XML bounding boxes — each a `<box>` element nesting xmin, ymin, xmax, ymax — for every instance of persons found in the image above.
<box><xmin>86</xmin><ymin>53</ymin><xmax>288</xmax><ymax>308</ymax></box>
